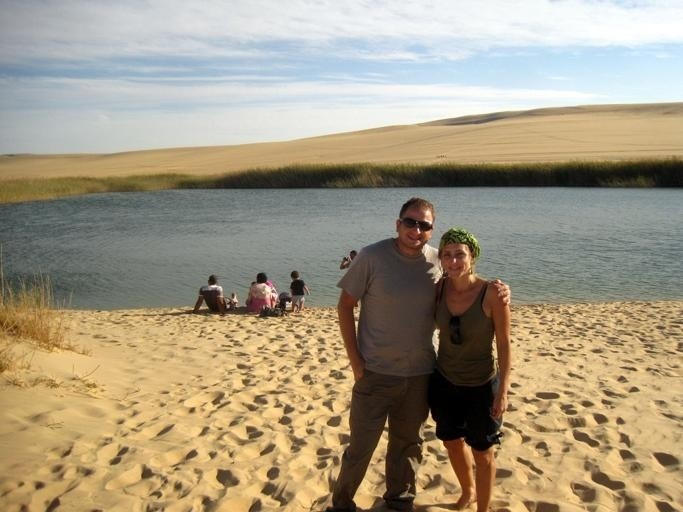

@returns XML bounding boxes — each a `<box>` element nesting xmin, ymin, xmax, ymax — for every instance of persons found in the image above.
<box><xmin>245</xmin><ymin>272</ymin><xmax>279</xmax><ymax>313</ymax></box>
<box><xmin>340</xmin><ymin>249</ymin><xmax>359</xmax><ymax>307</ymax></box>
<box><xmin>193</xmin><ymin>275</ymin><xmax>227</xmax><ymax>313</ymax></box>
<box><xmin>330</xmin><ymin>199</ymin><xmax>511</xmax><ymax>510</ymax></box>
<box><xmin>290</xmin><ymin>271</ymin><xmax>311</xmax><ymax>313</ymax></box>
<box><xmin>430</xmin><ymin>226</ymin><xmax>513</xmax><ymax>511</ymax></box>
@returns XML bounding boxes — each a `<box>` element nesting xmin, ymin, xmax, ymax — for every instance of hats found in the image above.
<box><xmin>439</xmin><ymin>226</ymin><xmax>480</xmax><ymax>259</ymax></box>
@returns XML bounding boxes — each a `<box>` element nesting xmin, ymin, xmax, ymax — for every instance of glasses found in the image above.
<box><xmin>449</xmin><ymin>315</ymin><xmax>463</xmax><ymax>347</ymax></box>
<box><xmin>397</xmin><ymin>216</ymin><xmax>434</xmax><ymax>232</ymax></box>
